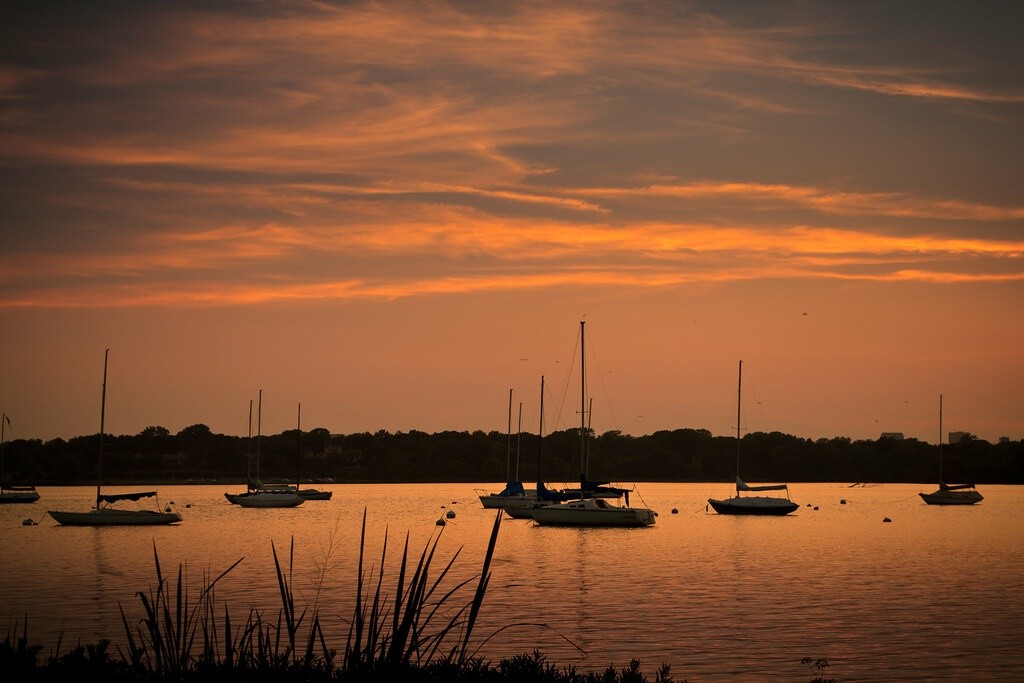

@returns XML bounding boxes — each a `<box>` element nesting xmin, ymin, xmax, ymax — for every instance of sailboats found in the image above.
<box><xmin>708</xmin><ymin>361</ymin><xmax>799</xmax><ymax>515</ymax></box>
<box><xmin>918</xmin><ymin>394</ymin><xmax>985</xmax><ymax>504</ymax></box>
<box><xmin>223</xmin><ymin>389</ymin><xmax>333</xmax><ymax>508</ymax></box>
<box><xmin>471</xmin><ymin>320</ymin><xmax>659</xmax><ymax>526</ymax></box>
<box><xmin>47</xmin><ymin>348</ymin><xmax>185</xmax><ymax>524</ymax></box>
<box><xmin>0</xmin><ymin>413</ymin><xmax>40</xmax><ymax>503</ymax></box>
